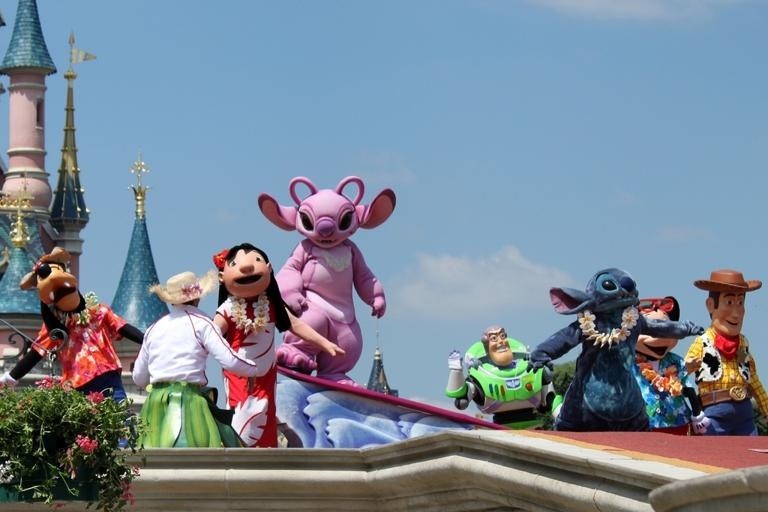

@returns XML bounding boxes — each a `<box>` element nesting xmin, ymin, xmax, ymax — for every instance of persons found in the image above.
<box><xmin>131</xmin><ymin>270</ymin><xmax>260</xmax><ymax>447</ymax></box>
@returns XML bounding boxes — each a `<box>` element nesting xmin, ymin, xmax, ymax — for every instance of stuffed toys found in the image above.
<box><xmin>442</xmin><ymin>324</ymin><xmax>566</xmax><ymax>431</ymax></box>
<box><xmin>522</xmin><ymin>267</ymin><xmax>709</xmax><ymax>430</ymax></box>
<box><xmin>682</xmin><ymin>269</ymin><xmax>768</xmax><ymax>436</ymax></box>
<box><xmin>0</xmin><ymin>247</ymin><xmax>149</xmax><ymax>450</ymax></box>
<box><xmin>256</xmin><ymin>175</ymin><xmax>397</xmax><ymax>391</ymax></box>
<box><xmin>206</xmin><ymin>242</ymin><xmax>348</xmax><ymax>450</ymax></box>
<box><xmin>623</xmin><ymin>292</ymin><xmax>712</xmax><ymax>437</ymax></box>
